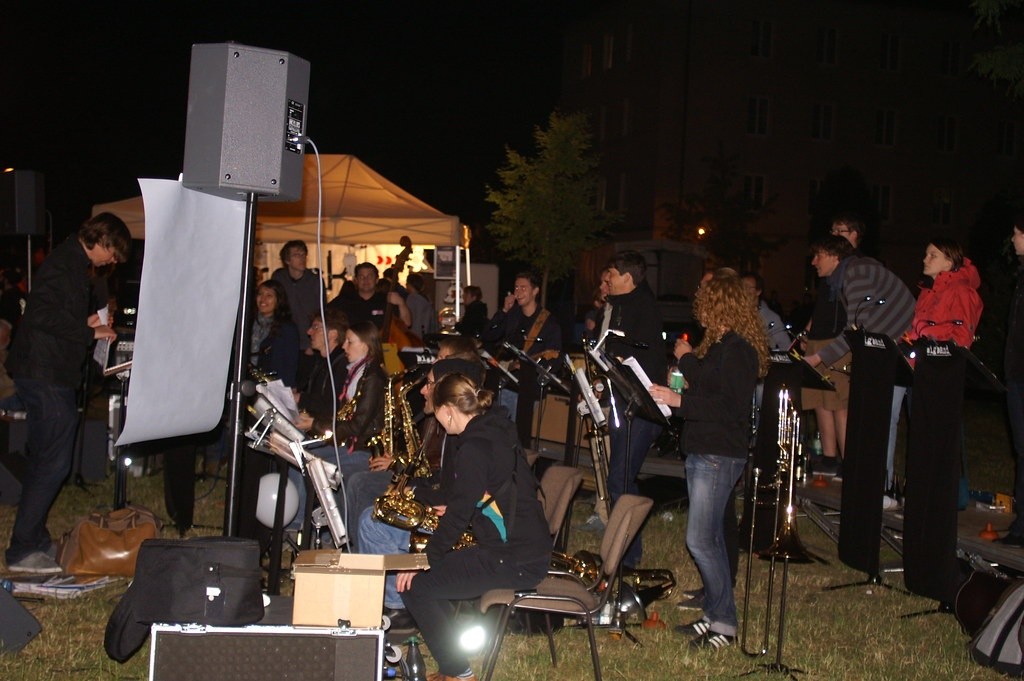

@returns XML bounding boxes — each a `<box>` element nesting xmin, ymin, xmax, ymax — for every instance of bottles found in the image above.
<box><xmin>666</xmin><ymin>333</ymin><xmax>689</xmax><ymax>395</ymax></box>
<box><xmin>406</xmin><ymin>635</ymin><xmax>427</xmax><ymax>681</ymax></box>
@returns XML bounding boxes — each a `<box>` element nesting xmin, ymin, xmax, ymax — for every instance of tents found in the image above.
<box><xmin>91</xmin><ymin>155</ymin><xmax>471</xmax><ymax>324</ymax></box>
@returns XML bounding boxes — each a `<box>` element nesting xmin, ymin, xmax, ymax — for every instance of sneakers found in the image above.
<box><xmin>576</xmin><ymin>512</ymin><xmax>604</xmax><ymax>530</ymax></box>
<box><xmin>674</xmin><ymin>619</ymin><xmax>710</xmax><ymax>635</ymax></box>
<box><xmin>383</xmin><ymin>609</ymin><xmax>415</xmax><ymax>634</ymax></box>
<box><xmin>8</xmin><ymin>550</ymin><xmax>63</xmax><ymax>573</ymax></box>
<box><xmin>690</xmin><ymin>629</ymin><xmax>738</xmax><ymax>649</ymax></box>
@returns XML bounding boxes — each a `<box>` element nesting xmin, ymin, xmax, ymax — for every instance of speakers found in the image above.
<box><xmin>0</xmin><ymin>170</ymin><xmax>46</xmax><ymax>236</ymax></box>
<box><xmin>182</xmin><ymin>43</ymin><xmax>311</xmax><ymax>205</ymax></box>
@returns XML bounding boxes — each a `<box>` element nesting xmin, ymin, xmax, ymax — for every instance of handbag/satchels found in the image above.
<box><xmin>55</xmin><ymin>505</ymin><xmax>157</xmax><ymax>577</ymax></box>
<box><xmin>104</xmin><ymin>536</ymin><xmax>265</xmax><ymax>664</ymax></box>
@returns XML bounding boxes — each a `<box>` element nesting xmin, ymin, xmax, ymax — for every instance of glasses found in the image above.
<box><xmin>829</xmin><ymin>229</ymin><xmax>849</xmax><ymax>236</ymax></box>
<box><xmin>426</xmin><ymin>377</ymin><xmax>435</xmax><ymax>391</ymax></box>
<box><xmin>311</xmin><ymin>324</ymin><xmax>322</xmax><ymax>331</ymax></box>
<box><xmin>745</xmin><ymin>284</ymin><xmax>755</xmax><ymax>290</ymax></box>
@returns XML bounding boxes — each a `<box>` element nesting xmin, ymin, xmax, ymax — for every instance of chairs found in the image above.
<box><xmin>453</xmin><ymin>449</ymin><xmax>654</xmax><ymax>681</ymax></box>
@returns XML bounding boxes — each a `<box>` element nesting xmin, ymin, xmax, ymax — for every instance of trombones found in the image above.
<box><xmin>741</xmin><ymin>379</ymin><xmax>816</xmax><ymax>658</ymax></box>
<box><xmin>582</xmin><ymin>330</ymin><xmax>610</xmax><ymax>522</ymax></box>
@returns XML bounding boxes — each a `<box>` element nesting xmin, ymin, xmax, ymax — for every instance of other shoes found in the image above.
<box><xmin>426</xmin><ymin>671</ymin><xmax>478</xmax><ymax>681</ymax></box>
<box><xmin>617</xmin><ymin>565</ymin><xmax>634</xmax><ymax>576</ymax></box>
<box><xmin>677</xmin><ymin>586</ymin><xmax>704</xmax><ymax>610</ymax></box>
<box><xmin>832</xmin><ymin>473</ymin><xmax>842</xmax><ymax>482</ymax></box>
<box><xmin>992</xmin><ymin>533</ymin><xmax>1024</xmax><ymax>548</ymax></box>
<box><xmin>895</xmin><ymin>511</ymin><xmax>903</xmax><ymax>519</ymax></box>
<box><xmin>812</xmin><ymin>461</ymin><xmax>837</xmax><ymax>476</ymax></box>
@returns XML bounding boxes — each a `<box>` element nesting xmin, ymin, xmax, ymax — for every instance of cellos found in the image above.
<box><xmin>380</xmin><ymin>236</ymin><xmax>425</xmax><ymax>399</ymax></box>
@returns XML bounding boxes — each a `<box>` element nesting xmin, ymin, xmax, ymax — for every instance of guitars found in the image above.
<box><xmin>498</xmin><ymin>349</ymin><xmax>561</xmax><ymax>389</ymax></box>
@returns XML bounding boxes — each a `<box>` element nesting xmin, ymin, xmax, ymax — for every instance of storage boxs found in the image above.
<box><xmin>289</xmin><ymin>549</ymin><xmax>430</xmax><ymax>628</ymax></box>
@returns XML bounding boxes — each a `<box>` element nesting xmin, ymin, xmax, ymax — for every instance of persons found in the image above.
<box><xmin>1</xmin><ymin>212</ymin><xmax>1024</xmax><ymax>681</ymax></box>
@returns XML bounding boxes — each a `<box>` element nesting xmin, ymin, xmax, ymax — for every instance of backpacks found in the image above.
<box><xmin>498</xmin><ymin>608</ymin><xmax>564</xmax><ymax>637</ymax></box>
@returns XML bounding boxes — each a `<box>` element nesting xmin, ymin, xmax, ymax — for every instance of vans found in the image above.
<box><xmin>531</xmin><ymin>228</ymin><xmax>793</xmax><ymax>352</ymax></box>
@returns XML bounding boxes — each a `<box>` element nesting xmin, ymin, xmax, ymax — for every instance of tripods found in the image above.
<box><xmin>66</xmin><ymin>359</ymin><xmax>103</xmax><ymax>499</ymax></box>
<box><xmin>562</xmin><ymin>355</ymin><xmax>672</xmax><ymax>650</ymax></box>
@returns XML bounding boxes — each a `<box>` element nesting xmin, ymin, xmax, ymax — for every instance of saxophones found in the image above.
<box><xmin>373</xmin><ymin>445</ymin><xmax>477</xmax><ymax>552</ymax></box>
<box><xmin>386</xmin><ymin>372</ymin><xmax>433</xmax><ymax>477</ymax></box>
<box><xmin>335</xmin><ymin>363</ymin><xmax>371</xmax><ymax>421</ymax></box>
<box><xmin>366</xmin><ymin>365</ymin><xmax>422</xmax><ymax>460</ymax></box>
<box><xmin>249</xmin><ymin>362</ymin><xmax>325</xmax><ymax>441</ymax></box>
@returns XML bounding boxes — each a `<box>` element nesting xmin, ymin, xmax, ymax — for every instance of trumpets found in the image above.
<box><xmin>786</xmin><ymin>325</ymin><xmax>810</xmax><ymax>345</ymax></box>
<box><xmin>831</xmin><ymin>361</ymin><xmax>852</xmax><ymax>376</ymax></box>
